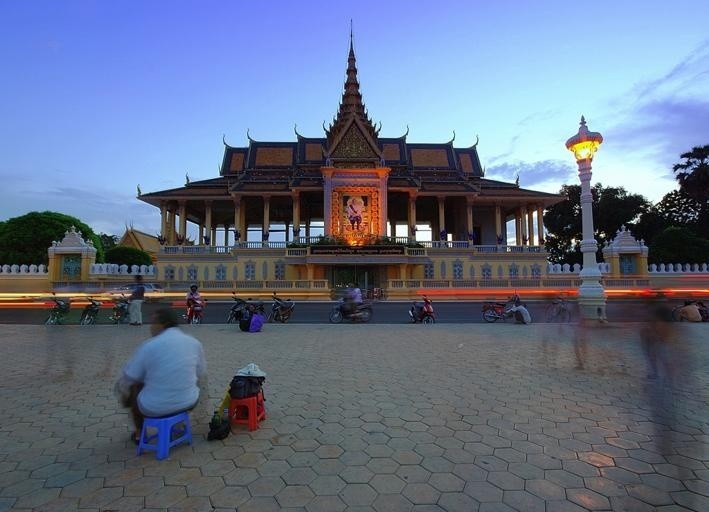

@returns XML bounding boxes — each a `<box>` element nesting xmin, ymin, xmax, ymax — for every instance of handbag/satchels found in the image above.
<box><xmin>239</xmin><ymin>314</ymin><xmax>266</xmax><ymax>332</ymax></box>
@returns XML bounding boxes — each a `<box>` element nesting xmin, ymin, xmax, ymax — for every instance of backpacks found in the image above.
<box><xmin>228</xmin><ymin>377</ymin><xmax>267</xmax><ymax>402</ymax></box>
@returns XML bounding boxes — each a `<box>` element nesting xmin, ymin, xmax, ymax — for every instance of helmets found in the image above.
<box><xmin>190</xmin><ymin>283</ymin><xmax>198</xmax><ymax>288</ymax></box>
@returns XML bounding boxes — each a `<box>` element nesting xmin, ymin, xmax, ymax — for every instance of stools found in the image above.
<box><xmin>138</xmin><ymin>412</ymin><xmax>194</xmax><ymax>460</ymax></box>
<box><xmin>228</xmin><ymin>393</ymin><xmax>267</xmax><ymax>432</ymax></box>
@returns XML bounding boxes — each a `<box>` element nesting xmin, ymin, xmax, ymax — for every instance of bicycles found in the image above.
<box><xmin>671</xmin><ymin>298</ymin><xmax>708</xmax><ymax>322</ymax></box>
<box><xmin>542</xmin><ymin>287</ymin><xmax>572</xmax><ymax>324</ymax></box>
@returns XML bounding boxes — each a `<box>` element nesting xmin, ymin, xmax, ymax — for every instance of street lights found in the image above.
<box><xmin>565</xmin><ymin>113</ymin><xmax>614</xmax><ymax>332</ymax></box>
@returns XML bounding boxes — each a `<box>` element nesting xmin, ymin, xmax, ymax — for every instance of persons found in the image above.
<box><xmin>342</xmin><ymin>282</ymin><xmax>364</xmax><ymax>318</ymax></box>
<box><xmin>504</xmin><ymin>301</ymin><xmax>532</xmax><ymax>325</ymax></box>
<box><xmin>238</xmin><ymin>303</ymin><xmax>265</xmax><ymax>332</ymax></box>
<box><xmin>347</xmin><ymin>199</ymin><xmax>362</xmax><ymax>231</ymax></box>
<box><xmin>676</xmin><ymin>300</ymin><xmax>709</xmax><ymax>322</ymax></box>
<box><xmin>118</xmin><ymin>306</ymin><xmax>208</xmax><ymax>446</ymax></box>
<box><xmin>126</xmin><ymin>275</ymin><xmax>145</xmax><ymax>326</ymax></box>
<box><xmin>186</xmin><ymin>283</ymin><xmax>205</xmax><ymax>321</ymax></box>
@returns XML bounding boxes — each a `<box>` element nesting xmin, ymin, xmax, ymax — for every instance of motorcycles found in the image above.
<box><xmin>407</xmin><ymin>294</ymin><xmax>437</xmax><ymax>325</ymax></box>
<box><xmin>80</xmin><ymin>296</ymin><xmax>105</xmax><ymax>327</ymax></box>
<box><xmin>267</xmin><ymin>290</ymin><xmax>299</xmax><ymax>323</ymax></box>
<box><xmin>180</xmin><ymin>297</ymin><xmax>209</xmax><ymax>325</ymax></box>
<box><xmin>479</xmin><ymin>290</ymin><xmax>536</xmax><ymax>325</ymax></box>
<box><xmin>41</xmin><ymin>292</ymin><xmax>75</xmax><ymax>326</ymax></box>
<box><xmin>329</xmin><ymin>296</ymin><xmax>374</xmax><ymax>324</ymax></box>
<box><xmin>226</xmin><ymin>290</ymin><xmax>266</xmax><ymax>326</ymax></box>
<box><xmin>110</xmin><ymin>291</ymin><xmax>137</xmax><ymax>326</ymax></box>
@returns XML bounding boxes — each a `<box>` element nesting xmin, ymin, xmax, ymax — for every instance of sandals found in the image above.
<box><xmin>131</xmin><ymin>432</ymin><xmax>148</xmax><ymax>446</ymax></box>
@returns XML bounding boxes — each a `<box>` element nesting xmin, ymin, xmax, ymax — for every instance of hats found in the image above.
<box><xmin>235</xmin><ymin>363</ymin><xmax>266</xmax><ymax>377</ymax></box>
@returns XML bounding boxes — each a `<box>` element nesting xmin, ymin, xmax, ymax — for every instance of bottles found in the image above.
<box><xmin>212</xmin><ymin>409</ymin><xmax>228</xmax><ymax>425</ymax></box>
